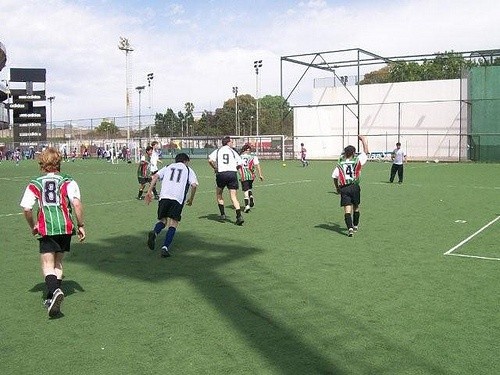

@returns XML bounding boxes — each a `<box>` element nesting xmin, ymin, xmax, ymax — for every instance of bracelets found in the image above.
<box><xmin>77</xmin><ymin>223</ymin><xmax>85</xmax><ymax>227</ymax></box>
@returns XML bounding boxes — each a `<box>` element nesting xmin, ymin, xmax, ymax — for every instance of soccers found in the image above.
<box><xmin>282</xmin><ymin>163</ymin><xmax>287</xmax><ymax>167</ymax></box>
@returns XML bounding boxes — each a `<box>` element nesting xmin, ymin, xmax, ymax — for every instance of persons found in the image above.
<box><xmin>144</xmin><ymin>152</ymin><xmax>198</xmax><ymax>259</ymax></box>
<box><xmin>70</xmin><ymin>148</ymin><xmax>77</xmax><ymax>163</ymax></box>
<box><xmin>237</xmin><ymin>144</ymin><xmax>264</xmax><ymax>213</ymax></box>
<box><xmin>96</xmin><ymin>146</ymin><xmax>132</xmax><ymax>163</ymax></box>
<box><xmin>386</xmin><ymin>142</ymin><xmax>408</xmax><ymax>185</ymax></box>
<box><xmin>331</xmin><ymin>135</ymin><xmax>370</xmax><ymax>236</ymax></box>
<box><xmin>81</xmin><ymin>147</ymin><xmax>88</xmax><ymax>160</ymax></box>
<box><xmin>19</xmin><ymin>147</ymin><xmax>86</xmax><ymax>317</ymax></box>
<box><xmin>300</xmin><ymin>142</ymin><xmax>310</xmax><ymax>168</ymax></box>
<box><xmin>62</xmin><ymin>146</ymin><xmax>70</xmax><ymax>163</ymax></box>
<box><xmin>0</xmin><ymin>145</ymin><xmax>45</xmax><ymax>163</ymax></box>
<box><xmin>208</xmin><ymin>134</ymin><xmax>245</xmax><ymax>226</ymax></box>
<box><xmin>137</xmin><ymin>140</ymin><xmax>164</xmax><ymax>201</ymax></box>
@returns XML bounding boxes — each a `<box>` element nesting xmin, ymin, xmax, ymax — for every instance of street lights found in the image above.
<box><xmin>233</xmin><ymin>87</ymin><xmax>238</xmax><ymax>135</ymax></box>
<box><xmin>147</xmin><ymin>72</ymin><xmax>154</xmax><ymax>147</ymax></box>
<box><xmin>253</xmin><ymin>60</ymin><xmax>262</xmax><ymax>136</ymax></box>
<box><xmin>118</xmin><ymin>36</ymin><xmax>134</xmax><ymax>149</ymax></box>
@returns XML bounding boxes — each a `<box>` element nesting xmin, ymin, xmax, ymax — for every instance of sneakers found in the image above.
<box><xmin>235</xmin><ymin>216</ymin><xmax>244</xmax><ymax>225</ymax></box>
<box><xmin>161</xmin><ymin>246</ymin><xmax>170</xmax><ymax>256</ymax></box>
<box><xmin>48</xmin><ymin>288</ymin><xmax>64</xmax><ymax>317</ymax></box>
<box><xmin>148</xmin><ymin>231</ymin><xmax>157</xmax><ymax>250</ymax></box>
<box><xmin>219</xmin><ymin>215</ymin><xmax>225</xmax><ymax>220</ymax></box>
<box><xmin>244</xmin><ymin>205</ymin><xmax>250</xmax><ymax>213</ymax></box>
<box><xmin>44</xmin><ymin>299</ymin><xmax>52</xmax><ymax>309</ymax></box>
<box><xmin>250</xmin><ymin>195</ymin><xmax>254</xmax><ymax>207</ymax></box>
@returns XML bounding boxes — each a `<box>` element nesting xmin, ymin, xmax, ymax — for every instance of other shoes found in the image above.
<box><xmin>353</xmin><ymin>226</ymin><xmax>358</xmax><ymax>231</ymax></box>
<box><xmin>138</xmin><ymin>197</ymin><xmax>143</xmax><ymax>200</ymax></box>
<box><xmin>399</xmin><ymin>181</ymin><xmax>401</xmax><ymax>183</ymax></box>
<box><xmin>348</xmin><ymin>229</ymin><xmax>353</xmax><ymax>237</ymax></box>
<box><xmin>155</xmin><ymin>196</ymin><xmax>159</xmax><ymax>199</ymax></box>
